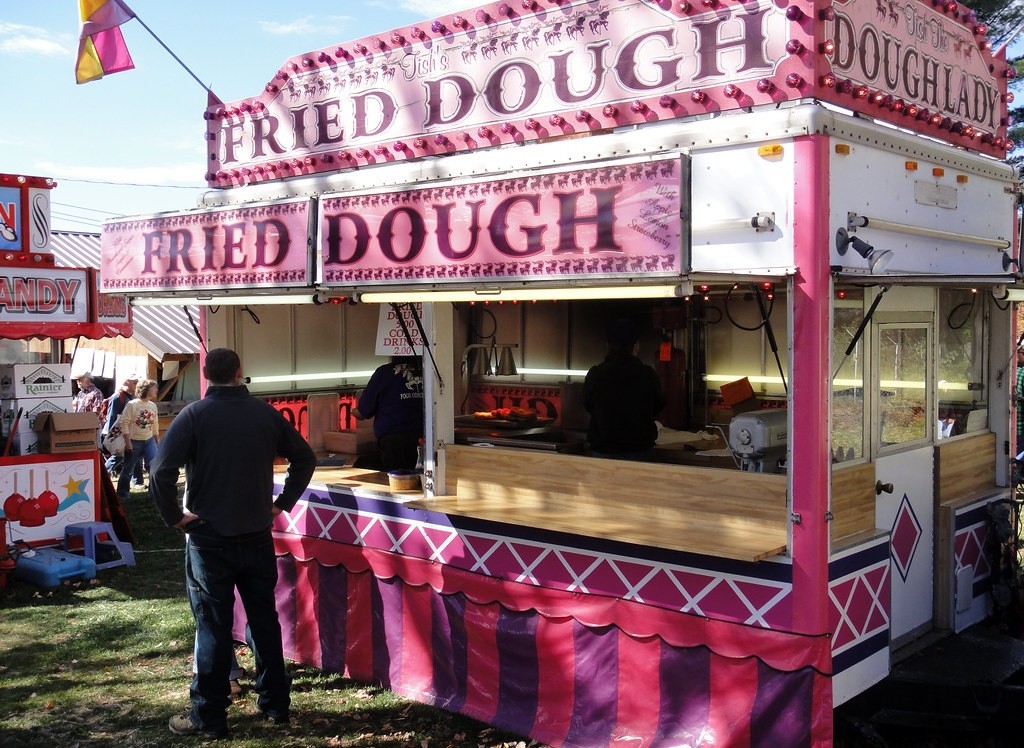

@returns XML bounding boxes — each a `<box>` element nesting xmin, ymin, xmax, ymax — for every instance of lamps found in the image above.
<box><xmin>460</xmin><ymin>342</ymin><xmax>521</xmax><ymax>377</ymax></box>
<box><xmin>845</xmin><ymin>211</ymin><xmax>1011</xmax><ymax>253</ymax></box>
<box><xmin>836</xmin><ymin>226</ymin><xmax>895</xmax><ymax>276</ymax></box>
<box><xmin>690</xmin><ymin>211</ymin><xmax>775</xmax><ymax>233</ymax></box>
<box><xmin>993</xmin><ymin>284</ymin><xmax>1024</xmax><ymax>302</ymax></box>
<box><xmin>124</xmin><ymin>282</ymin><xmax>697</xmax><ymax>307</ymax></box>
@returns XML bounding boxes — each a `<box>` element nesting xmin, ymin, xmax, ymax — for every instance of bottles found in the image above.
<box><xmin>415</xmin><ymin>438</ymin><xmax>424</xmax><ymax>469</ymax></box>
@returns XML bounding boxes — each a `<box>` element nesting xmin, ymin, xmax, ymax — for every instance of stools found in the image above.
<box><xmin>64</xmin><ymin>521</ymin><xmax>136</xmax><ymax>571</ymax></box>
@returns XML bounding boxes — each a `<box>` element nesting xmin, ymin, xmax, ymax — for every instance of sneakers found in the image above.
<box><xmin>168</xmin><ymin>713</ymin><xmax>215</xmax><ymax>737</ymax></box>
<box><xmin>255</xmin><ymin>697</ymin><xmax>290</xmax><ymax>725</ymax></box>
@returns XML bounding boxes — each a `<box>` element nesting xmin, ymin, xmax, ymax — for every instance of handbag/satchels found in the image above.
<box><xmin>103</xmin><ymin>401</ymin><xmax>135</xmax><ymax>455</ymax></box>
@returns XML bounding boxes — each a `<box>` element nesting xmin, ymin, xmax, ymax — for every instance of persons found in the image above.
<box><xmin>352</xmin><ymin>357</ymin><xmax>424</xmax><ymax>472</ymax></box>
<box><xmin>580</xmin><ymin>319</ymin><xmax>665</xmax><ymax>460</ymax></box>
<box><xmin>1017</xmin><ymin>340</ymin><xmax>1024</xmax><ymax>463</ymax></box>
<box><xmin>71</xmin><ymin>371</ymin><xmax>103</xmax><ymax>449</ymax></box>
<box><xmin>116</xmin><ymin>379</ymin><xmax>159</xmax><ymax>499</ymax></box>
<box><xmin>104</xmin><ymin>376</ymin><xmax>147</xmax><ymax>489</ymax></box>
<box><xmin>149</xmin><ymin>348</ymin><xmax>316</xmax><ymax>735</ymax></box>
<box><xmin>229</xmin><ymin>622</ymin><xmax>257</xmax><ymax>693</ymax></box>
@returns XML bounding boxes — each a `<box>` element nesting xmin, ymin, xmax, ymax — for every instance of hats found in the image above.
<box><xmin>74</xmin><ymin>370</ymin><xmax>95</xmax><ymax>379</ymax></box>
<box><xmin>123</xmin><ymin>373</ymin><xmax>142</xmax><ymax>385</ymax></box>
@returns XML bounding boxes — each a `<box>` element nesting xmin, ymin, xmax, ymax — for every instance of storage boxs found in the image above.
<box><xmin>323</xmin><ymin>425</ymin><xmax>375</xmax><ymax>455</ymax></box>
<box><xmin>0</xmin><ymin>362</ymin><xmax>102</xmax><ymax>457</ymax></box>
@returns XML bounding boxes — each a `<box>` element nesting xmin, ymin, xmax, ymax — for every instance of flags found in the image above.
<box><xmin>75</xmin><ymin>0</ymin><xmax>136</xmax><ymax>85</ymax></box>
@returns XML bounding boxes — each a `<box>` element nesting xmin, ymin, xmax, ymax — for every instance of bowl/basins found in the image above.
<box><xmin>388</xmin><ymin>470</ymin><xmax>422</xmax><ymax>490</ymax></box>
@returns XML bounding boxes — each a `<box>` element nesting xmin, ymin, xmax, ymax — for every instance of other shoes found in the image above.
<box><xmin>228</xmin><ymin>679</ymin><xmax>242</xmax><ymax>698</ymax></box>
<box><xmin>119</xmin><ymin>491</ymin><xmax>130</xmax><ymax>498</ymax></box>
<box><xmin>128</xmin><ymin>483</ymin><xmax>147</xmax><ymax>489</ymax></box>
